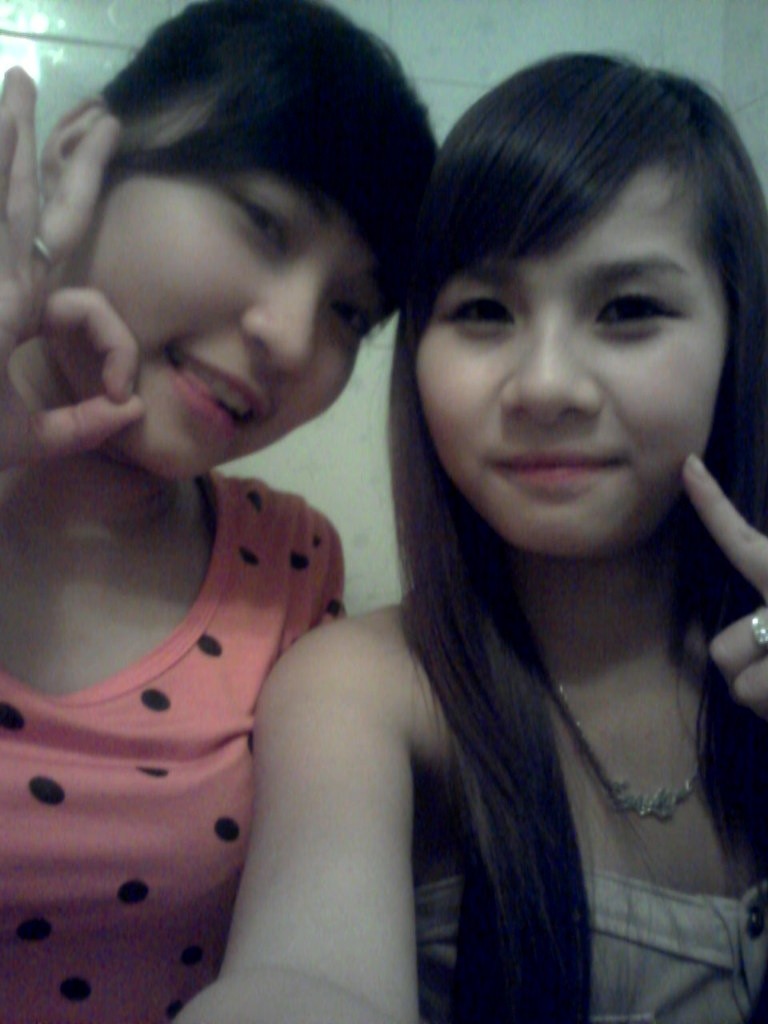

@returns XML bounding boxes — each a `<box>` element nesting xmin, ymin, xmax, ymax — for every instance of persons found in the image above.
<box><xmin>0</xmin><ymin>0</ymin><xmax>437</xmax><ymax>1024</ymax></box>
<box><xmin>170</xmin><ymin>54</ymin><xmax>768</xmax><ymax>1024</ymax></box>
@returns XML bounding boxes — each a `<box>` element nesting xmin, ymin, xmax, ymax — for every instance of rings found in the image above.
<box><xmin>34</xmin><ymin>236</ymin><xmax>52</xmax><ymax>260</ymax></box>
<box><xmin>751</xmin><ymin>607</ymin><xmax>768</xmax><ymax>645</ymax></box>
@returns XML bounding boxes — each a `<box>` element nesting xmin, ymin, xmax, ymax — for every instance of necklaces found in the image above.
<box><xmin>544</xmin><ymin>675</ymin><xmax>697</xmax><ymax>822</ymax></box>
